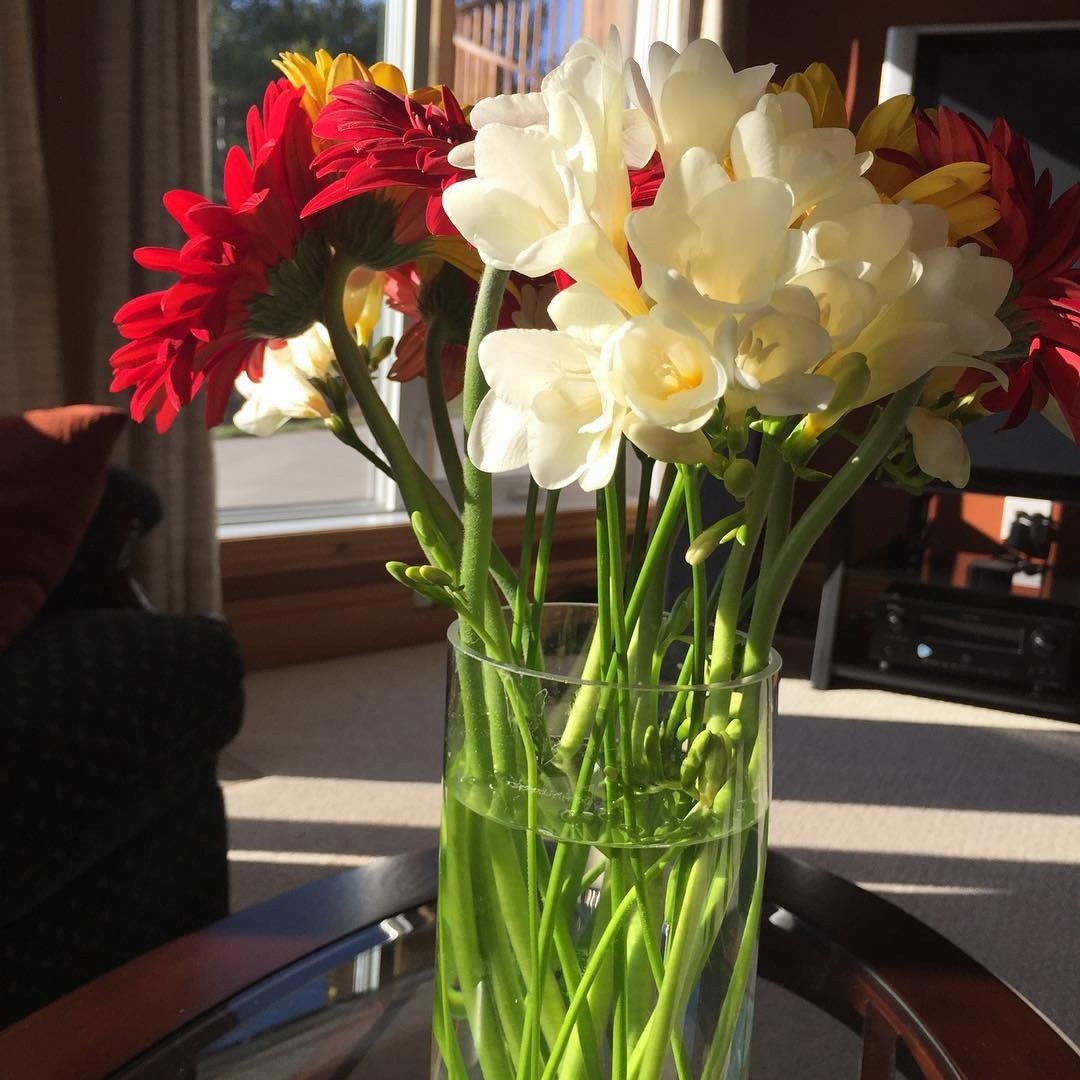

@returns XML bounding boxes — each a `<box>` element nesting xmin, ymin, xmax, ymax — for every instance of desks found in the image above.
<box><xmin>0</xmin><ymin>851</ymin><xmax>1078</xmax><ymax>1080</ymax></box>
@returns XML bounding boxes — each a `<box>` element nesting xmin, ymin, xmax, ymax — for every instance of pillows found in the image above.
<box><xmin>0</xmin><ymin>402</ymin><xmax>127</xmax><ymax>649</ymax></box>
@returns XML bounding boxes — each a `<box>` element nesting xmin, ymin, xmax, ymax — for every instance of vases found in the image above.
<box><xmin>429</xmin><ymin>605</ymin><xmax>781</xmax><ymax>1080</ymax></box>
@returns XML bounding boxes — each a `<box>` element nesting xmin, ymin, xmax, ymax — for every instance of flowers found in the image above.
<box><xmin>106</xmin><ymin>24</ymin><xmax>1080</xmax><ymax>1080</ymax></box>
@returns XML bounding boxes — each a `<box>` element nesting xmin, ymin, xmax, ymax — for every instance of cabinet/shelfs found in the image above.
<box><xmin>809</xmin><ymin>467</ymin><xmax>1080</xmax><ymax>724</ymax></box>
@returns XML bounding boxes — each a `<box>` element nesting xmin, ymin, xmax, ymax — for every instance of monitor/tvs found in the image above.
<box><xmin>876</xmin><ymin>18</ymin><xmax>1080</xmax><ymax>272</ymax></box>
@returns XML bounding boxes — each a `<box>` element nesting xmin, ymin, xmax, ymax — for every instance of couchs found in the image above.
<box><xmin>0</xmin><ymin>462</ymin><xmax>248</xmax><ymax>1031</ymax></box>
